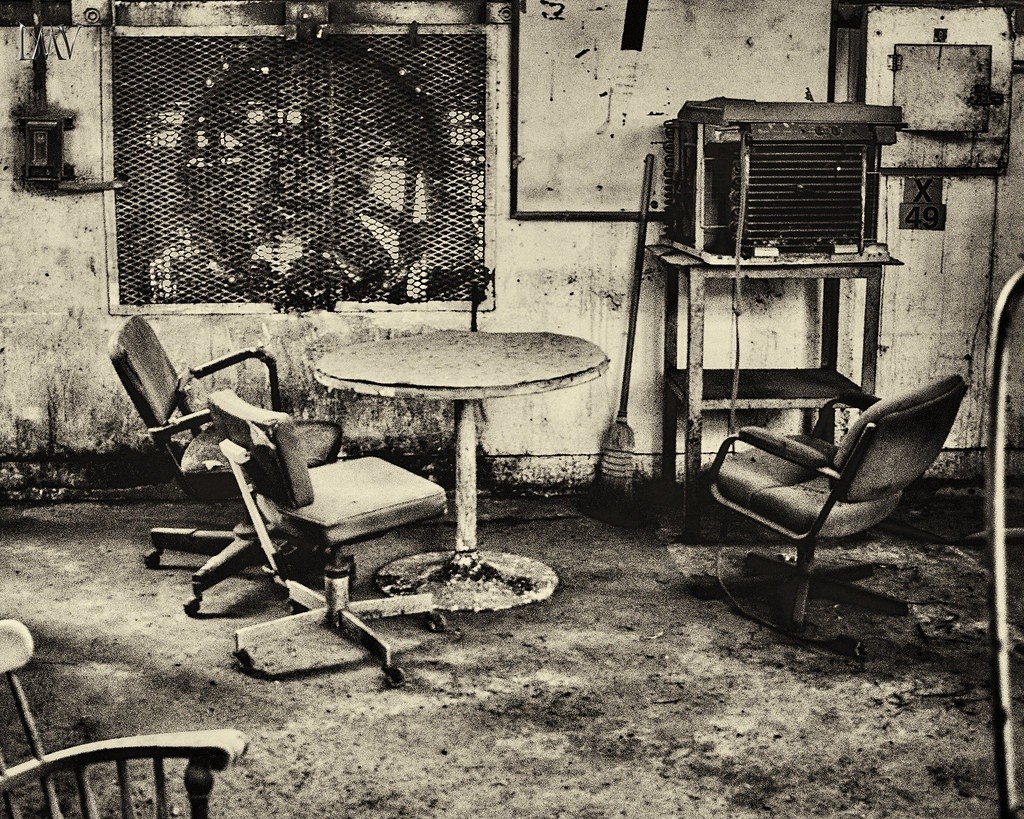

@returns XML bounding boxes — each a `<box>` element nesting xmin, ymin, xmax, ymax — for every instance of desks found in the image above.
<box><xmin>642</xmin><ymin>244</ymin><xmax>906</xmax><ymax>526</ymax></box>
<box><xmin>316</xmin><ymin>331</ymin><xmax>612</xmax><ymax>610</ymax></box>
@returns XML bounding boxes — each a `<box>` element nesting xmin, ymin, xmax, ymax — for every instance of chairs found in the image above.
<box><xmin>0</xmin><ymin>618</ymin><xmax>252</xmax><ymax>819</ymax></box>
<box><xmin>703</xmin><ymin>372</ymin><xmax>971</xmax><ymax>652</ymax></box>
<box><xmin>205</xmin><ymin>388</ymin><xmax>450</xmax><ymax>688</ymax></box>
<box><xmin>105</xmin><ymin>312</ymin><xmax>357</xmax><ymax>617</ymax></box>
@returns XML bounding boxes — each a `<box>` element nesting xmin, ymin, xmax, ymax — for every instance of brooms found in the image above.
<box><xmin>579</xmin><ymin>153</ymin><xmax>656</xmax><ymax>529</ymax></box>
